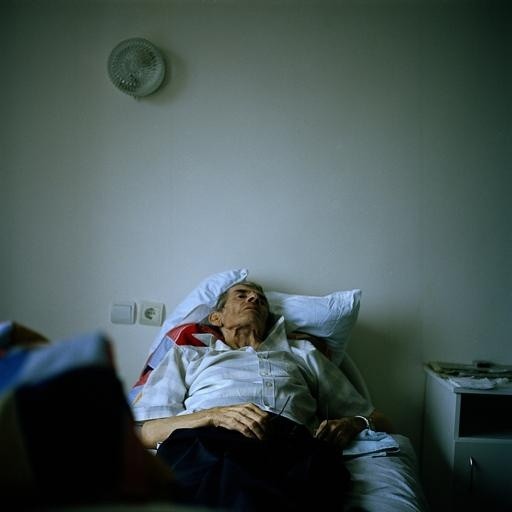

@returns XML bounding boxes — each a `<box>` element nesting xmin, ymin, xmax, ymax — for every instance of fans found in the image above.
<box><xmin>107</xmin><ymin>38</ymin><xmax>166</xmax><ymax>99</ymax></box>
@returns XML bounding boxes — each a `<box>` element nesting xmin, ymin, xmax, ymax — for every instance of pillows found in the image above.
<box><xmin>149</xmin><ymin>266</ymin><xmax>362</xmax><ymax>367</ymax></box>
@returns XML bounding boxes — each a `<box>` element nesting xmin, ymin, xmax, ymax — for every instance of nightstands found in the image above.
<box><xmin>423</xmin><ymin>363</ymin><xmax>511</xmax><ymax>505</ymax></box>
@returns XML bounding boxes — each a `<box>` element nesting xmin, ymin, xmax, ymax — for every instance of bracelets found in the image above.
<box><xmin>353</xmin><ymin>412</ymin><xmax>378</xmax><ymax>433</ymax></box>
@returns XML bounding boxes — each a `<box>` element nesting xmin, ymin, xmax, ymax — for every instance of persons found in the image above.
<box><xmin>131</xmin><ymin>280</ymin><xmax>396</xmax><ymax>512</ymax></box>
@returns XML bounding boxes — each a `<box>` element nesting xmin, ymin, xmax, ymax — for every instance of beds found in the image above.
<box><xmin>292</xmin><ymin>331</ymin><xmax>421</xmax><ymax>511</ymax></box>
